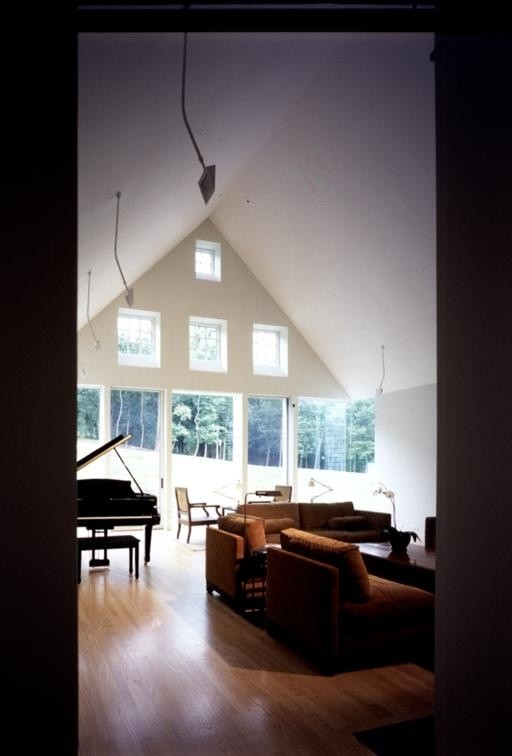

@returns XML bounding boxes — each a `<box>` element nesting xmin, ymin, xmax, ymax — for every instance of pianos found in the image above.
<box><xmin>76</xmin><ymin>433</ymin><xmax>160</xmax><ymax>566</ymax></box>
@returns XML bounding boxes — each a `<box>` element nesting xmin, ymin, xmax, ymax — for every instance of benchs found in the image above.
<box><xmin>77</xmin><ymin>534</ymin><xmax>143</xmax><ymax>584</ymax></box>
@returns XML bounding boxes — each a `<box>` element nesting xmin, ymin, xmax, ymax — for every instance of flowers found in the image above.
<box><xmin>374</xmin><ymin>487</ymin><xmax>396</xmax><ymax>530</ymax></box>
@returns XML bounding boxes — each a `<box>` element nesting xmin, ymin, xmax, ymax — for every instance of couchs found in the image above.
<box><xmin>207</xmin><ymin>503</ymin><xmax>437</xmax><ymax>676</ymax></box>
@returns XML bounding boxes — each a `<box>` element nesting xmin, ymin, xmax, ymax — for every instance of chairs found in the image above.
<box><xmin>174</xmin><ymin>485</ymin><xmax>295</xmax><ymax>544</ymax></box>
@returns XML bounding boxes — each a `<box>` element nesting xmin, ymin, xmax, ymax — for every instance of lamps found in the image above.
<box><xmin>114</xmin><ymin>195</ymin><xmax>135</xmax><ymax>308</ymax></box>
<box><xmin>179</xmin><ymin>36</ymin><xmax>216</xmax><ymax>204</ymax></box>
<box><xmin>374</xmin><ymin>345</ymin><xmax>388</xmax><ymax>398</ymax></box>
<box><xmin>86</xmin><ymin>271</ymin><xmax>103</xmax><ymax>351</ymax></box>
<box><xmin>303</xmin><ymin>478</ymin><xmax>336</xmax><ymax>505</ymax></box>
